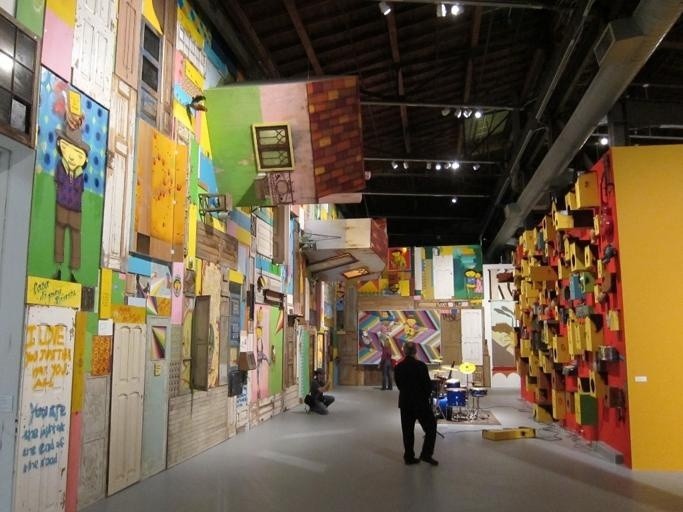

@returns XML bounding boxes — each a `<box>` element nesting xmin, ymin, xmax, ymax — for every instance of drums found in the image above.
<box><xmin>430</xmin><ymin>379</ymin><xmax>445</xmax><ymax>393</ymax></box>
<box><xmin>446</xmin><ymin>379</ymin><xmax>460</xmax><ymax>387</ymax></box>
<box><xmin>433</xmin><ymin>395</ymin><xmax>452</xmax><ymax>420</ymax></box>
<box><xmin>446</xmin><ymin>388</ymin><xmax>467</xmax><ymax>406</ymax></box>
<box><xmin>470</xmin><ymin>388</ymin><xmax>487</xmax><ymax>397</ymax></box>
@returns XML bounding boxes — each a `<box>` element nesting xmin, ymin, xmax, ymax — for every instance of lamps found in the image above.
<box><xmin>378</xmin><ymin>1</ymin><xmax>392</xmax><ymax>17</ymax></box>
<box><xmin>440</xmin><ymin>106</ymin><xmax>485</xmax><ymax>121</ymax></box>
<box><xmin>435</xmin><ymin>3</ymin><xmax>466</xmax><ymax>18</ymax></box>
<box><xmin>390</xmin><ymin>160</ymin><xmax>482</xmax><ymax>172</ymax></box>
<box><xmin>451</xmin><ymin>196</ymin><xmax>459</xmax><ymax>203</ymax></box>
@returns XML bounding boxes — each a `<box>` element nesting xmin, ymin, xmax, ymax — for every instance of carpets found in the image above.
<box><xmin>416</xmin><ymin>406</ymin><xmax>503</xmax><ymax>426</ymax></box>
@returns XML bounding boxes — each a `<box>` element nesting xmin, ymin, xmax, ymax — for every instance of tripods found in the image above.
<box><xmin>451</xmin><ymin>374</ymin><xmax>490</xmax><ymax>423</ymax></box>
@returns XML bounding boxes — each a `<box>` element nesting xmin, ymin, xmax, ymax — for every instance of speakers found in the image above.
<box><xmin>592</xmin><ymin>18</ymin><xmax>646</xmax><ymax>65</ymax></box>
<box><xmin>504</xmin><ymin>202</ymin><xmax>521</xmax><ymax>218</ymax></box>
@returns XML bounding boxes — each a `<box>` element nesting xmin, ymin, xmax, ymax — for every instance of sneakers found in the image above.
<box><xmin>403</xmin><ymin>456</ymin><xmax>420</xmax><ymax>465</ymax></box>
<box><xmin>417</xmin><ymin>452</ymin><xmax>439</xmax><ymax>465</ymax></box>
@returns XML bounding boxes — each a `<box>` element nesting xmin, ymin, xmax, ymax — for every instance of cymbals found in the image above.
<box><xmin>441</xmin><ymin>365</ymin><xmax>460</xmax><ymax>371</ymax></box>
<box><xmin>460</xmin><ymin>363</ymin><xmax>475</xmax><ymax>373</ymax></box>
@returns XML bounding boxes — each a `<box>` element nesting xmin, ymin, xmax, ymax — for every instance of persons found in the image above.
<box><xmin>379</xmin><ymin>338</ymin><xmax>392</xmax><ymax>390</ymax></box>
<box><xmin>309</xmin><ymin>367</ymin><xmax>335</xmax><ymax>415</ymax></box>
<box><xmin>394</xmin><ymin>342</ymin><xmax>439</xmax><ymax>466</ymax></box>
